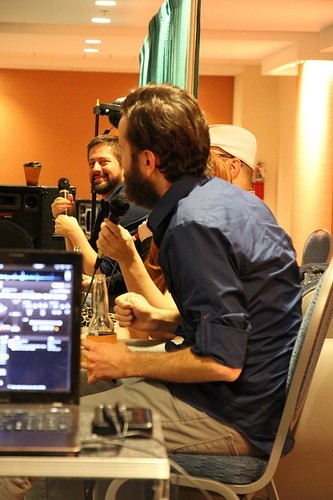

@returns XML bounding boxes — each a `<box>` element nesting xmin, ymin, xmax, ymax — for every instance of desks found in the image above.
<box><xmin>0</xmin><ymin>318</ymin><xmax>170</xmax><ymax>500</ymax></box>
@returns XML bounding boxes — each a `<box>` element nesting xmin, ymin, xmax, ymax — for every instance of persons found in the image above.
<box><xmin>0</xmin><ymin>84</ymin><xmax>303</xmax><ymax>500</ymax></box>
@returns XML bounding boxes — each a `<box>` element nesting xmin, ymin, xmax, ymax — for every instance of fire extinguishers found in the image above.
<box><xmin>252</xmin><ymin>160</ymin><xmax>265</xmax><ymax>200</ymax></box>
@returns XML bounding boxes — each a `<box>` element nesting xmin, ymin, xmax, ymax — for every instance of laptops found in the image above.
<box><xmin>0</xmin><ymin>249</ymin><xmax>84</xmax><ymax>454</ymax></box>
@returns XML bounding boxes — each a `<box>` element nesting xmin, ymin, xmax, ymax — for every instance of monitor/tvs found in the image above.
<box><xmin>0</xmin><ymin>185</ymin><xmax>75</xmax><ymax>250</ymax></box>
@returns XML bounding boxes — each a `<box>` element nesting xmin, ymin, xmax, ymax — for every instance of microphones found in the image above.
<box><xmin>58</xmin><ymin>177</ymin><xmax>70</xmax><ymax>215</ymax></box>
<box><xmin>95</xmin><ymin>192</ymin><xmax>129</xmax><ymax>272</ymax></box>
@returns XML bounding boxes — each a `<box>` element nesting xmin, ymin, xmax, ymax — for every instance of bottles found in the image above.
<box><xmin>87</xmin><ymin>272</ymin><xmax>118</xmax><ymax>383</ymax></box>
<box><xmin>73</xmin><ymin>245</ymin><xmax>80</xmax><ymax>252</ymax></box>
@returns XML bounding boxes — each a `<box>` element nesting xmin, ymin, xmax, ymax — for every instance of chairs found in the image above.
<box><xmin>85</xmin><ymin>229</ymin><xmax>333</xmax><ymax>500</ymax></box>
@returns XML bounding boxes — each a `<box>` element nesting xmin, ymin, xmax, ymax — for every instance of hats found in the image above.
<box><xmin>209</xmin><ymin>124</ymin><xmax>257</xmax><ymax>170</ymax></box>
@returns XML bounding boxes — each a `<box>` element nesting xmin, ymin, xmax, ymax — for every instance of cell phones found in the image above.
<box><xmin>119</xmin><ymin>404</ymin><xmax>152</xmax><ymax>432</ymax></box>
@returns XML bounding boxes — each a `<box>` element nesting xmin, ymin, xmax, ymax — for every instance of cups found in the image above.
<box><xmin>23</xmin><ymin>162</ymin><xmax>43</xmax><ymax>186</ymax></box>
